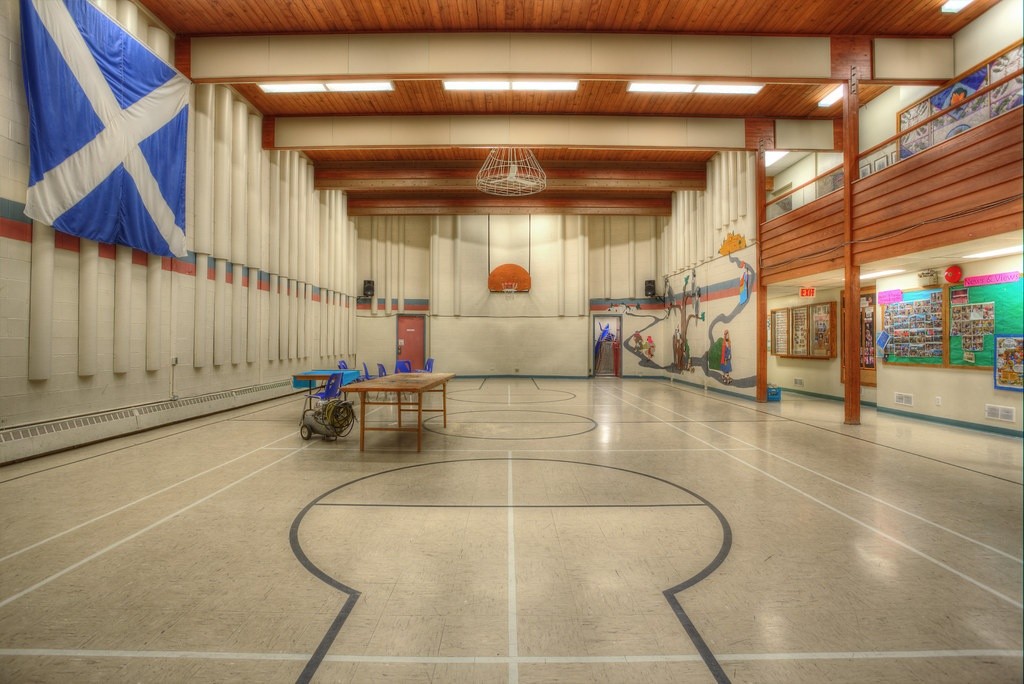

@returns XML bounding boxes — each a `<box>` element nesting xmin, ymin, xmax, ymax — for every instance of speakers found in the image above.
<box><xmin>645</xmin><ymin>280</ymin><xmax>655</xmax><ymax>297</ymax></box>
<box><xmin>364</xmin><ymin>279</ymin><xmax>374</xmax><ymax>297</ymax></box>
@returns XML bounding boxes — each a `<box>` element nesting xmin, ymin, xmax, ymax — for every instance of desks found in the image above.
<box><xmin>291</xmin><ymin>369</ymin><xmax>361</xmax><ymax>410</ymax></box>
<box><xmin>339</xmin><ymin>372</ymin><xmax>457</xmax><ymax>453</ymax></box>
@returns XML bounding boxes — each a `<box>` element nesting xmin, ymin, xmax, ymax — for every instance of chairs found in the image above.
<box><xmin>338</xmin><ymin>360</ymin><xmax>412</xmax><ymax>401</ymax></box>
<box><xmin>414</xmin><ymin>358</ymin><xmax>434</xmax><ymax>373</ymax></box>
<box><xmin>299</xmin><ymin>372</ymin><xmax>344</xmax><ymax>426</ymax></box>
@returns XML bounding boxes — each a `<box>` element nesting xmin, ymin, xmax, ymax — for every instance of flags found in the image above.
<box><xmin>19</xmin><ymin>0</ymin><xmax>192</xmax><ymax>257</ymax></box>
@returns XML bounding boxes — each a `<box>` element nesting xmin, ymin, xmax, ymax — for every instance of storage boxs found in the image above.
<box><xmin>768</xmin><ymin>386</ymin><xmax>781</xmax><ymax>402</ymax></box>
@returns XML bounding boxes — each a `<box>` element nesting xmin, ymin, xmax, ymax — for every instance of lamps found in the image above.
<box><xmin>364</xmin><ymin>280</ymin><xmax>375</xmax><ymax>298</ymax></box>
<box><xmin>644</xmin><ymin>280</ymin><xmax>657</xmax><ymax>298</ymax></box>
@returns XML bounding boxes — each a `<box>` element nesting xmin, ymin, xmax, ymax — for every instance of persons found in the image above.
<box><xmin>867</xmin><ymin>295</ymin><xmax>872</xmax><ymax>304</ymax></box>
